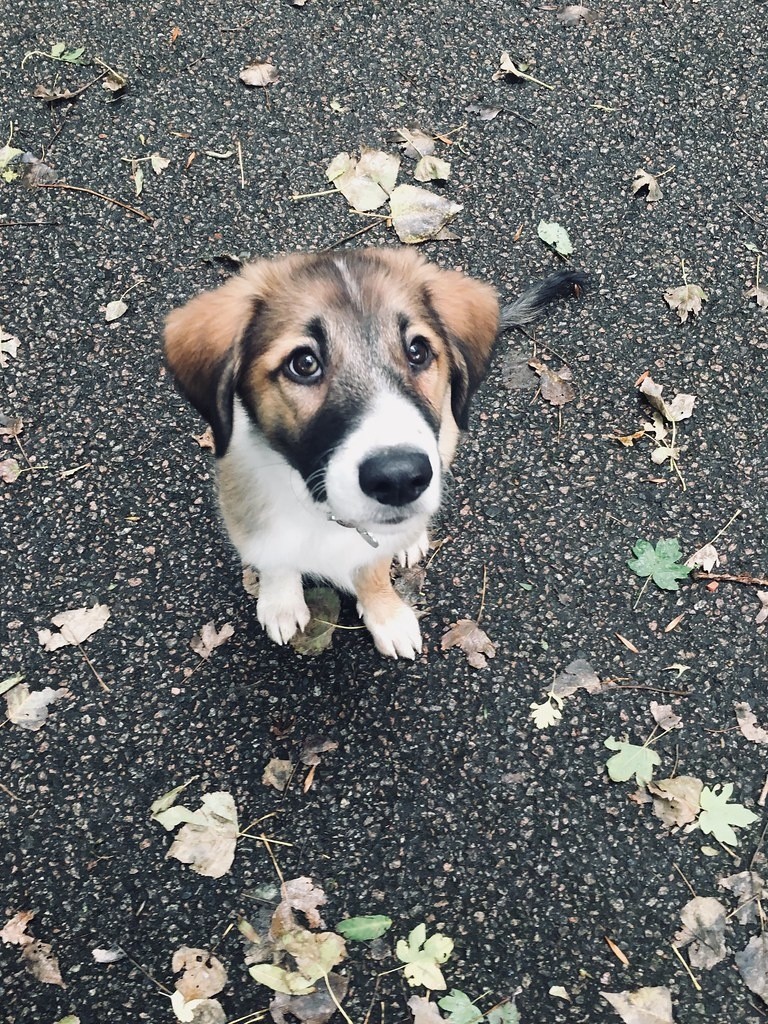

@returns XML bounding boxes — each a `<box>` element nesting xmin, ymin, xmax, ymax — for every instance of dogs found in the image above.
<box><xmin>157</xmin><ymin>245</ymin><xmax>597</xmax><ymax>663</ymax></box>
